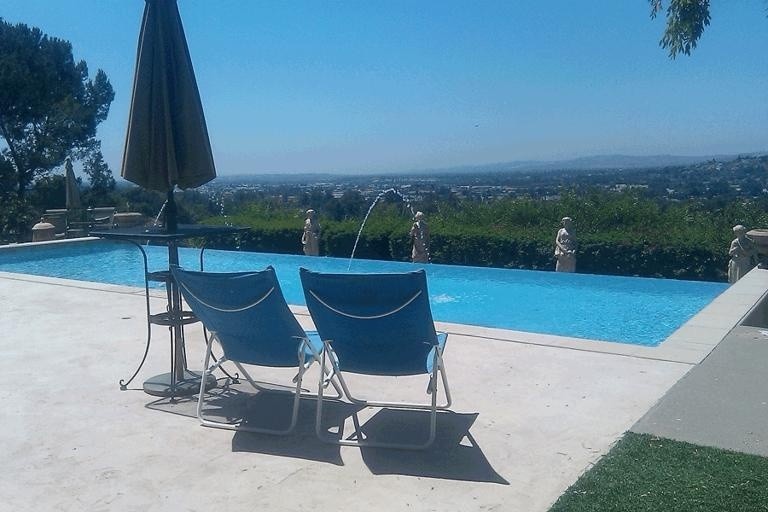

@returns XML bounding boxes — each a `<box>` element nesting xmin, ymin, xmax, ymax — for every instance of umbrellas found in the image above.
<box><xmin>63</xmin><ymin>156</ymin><xmax>82</xmax><ymax>212</ymax></box>
<box><xmin>116</xmin><ymin>1</ymin><xmax>219</xmax><ymax>380</ymax></box>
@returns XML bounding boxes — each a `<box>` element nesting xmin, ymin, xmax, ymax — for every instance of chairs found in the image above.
<box><xmin>300</xmin><ymin>266</ymin><xmax>451</xmax><ymax>450</ymax></box>
<box><xmin>170</xmin><ymin>263</ymin><xmax>342</xmax><ymax>436</ymax></box>
<box><xmin>44</xmin><ymin>208</ymin><xmax>117</xmax><ymax>238</ymax></box>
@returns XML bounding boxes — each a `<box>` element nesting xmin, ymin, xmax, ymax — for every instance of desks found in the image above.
<box><xmin>88</xmin><ymin>225</ymin><xmax>253</xmax><ymax>407</ymax></box>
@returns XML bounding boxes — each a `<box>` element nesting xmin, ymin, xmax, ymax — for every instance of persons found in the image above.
<box><xmin>726</xmin><ymin>224</ymin><xmax>756</xmax><ymax>284</ymax></box>
<box><xmin>408</xmin><ymin>210</ymin><xmax>431</xmax><ymax>264</ymax></box>
<box><xmin>553</xmin><ymin>216</ymin><xmax>577</xmax><ymax>272</ymax></box>
<box><xmin>300</xmin><ymin>209</ymin><xmax>324</xmax><ymax>256</ymax></box>
